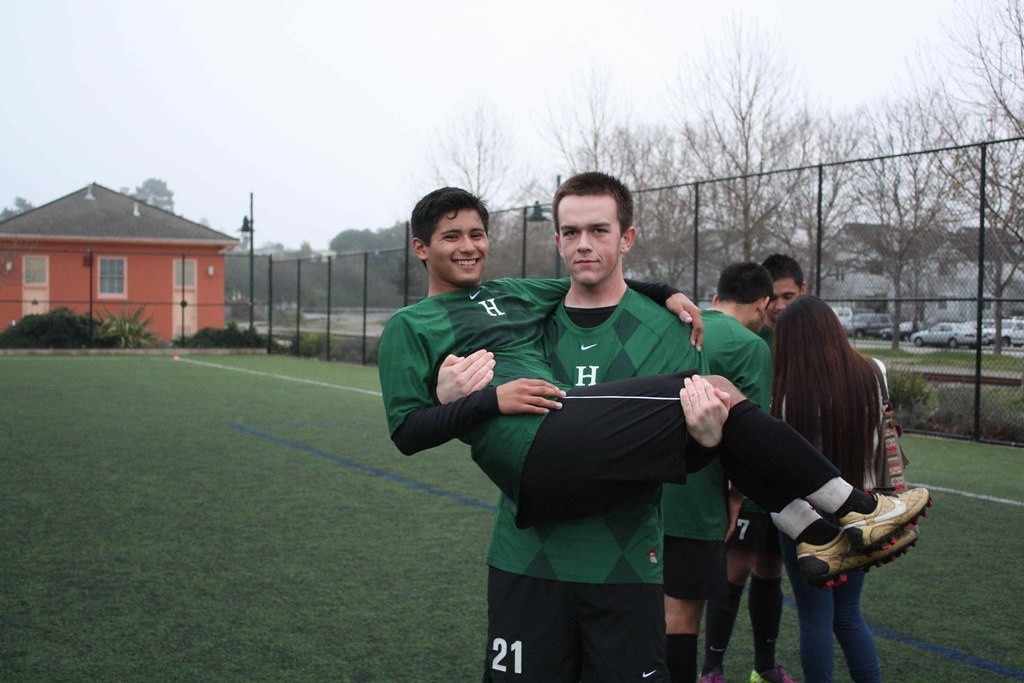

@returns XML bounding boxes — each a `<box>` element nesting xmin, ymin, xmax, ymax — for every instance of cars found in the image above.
<box><xmin>910</xmin><ymin>322</ymin><xmax>978</xmax><ymax>349</ymax></box>
<box><xmin>881</xmin><ymin>322</ymin><xmax>926</xmax><ymax>342</ymax></box>
<box><xmin>967</xmin><ymin>319</ymin><xmax>1024</xmax><ymax>348</ymax></box>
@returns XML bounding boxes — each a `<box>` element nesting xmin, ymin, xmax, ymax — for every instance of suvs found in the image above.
<box><xmin>845</xmin><ymin>313</ymin><xmax>906</xmax><ymax>339</ymax></box>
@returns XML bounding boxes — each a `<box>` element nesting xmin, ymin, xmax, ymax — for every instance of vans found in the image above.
<box><xmin>832</xmin><ymin>306</ymin><xmax>875</xmax><ymax>334</ymax></box>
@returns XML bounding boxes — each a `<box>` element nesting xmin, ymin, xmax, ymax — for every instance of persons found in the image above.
<box><xmin>698</xmin><ymin>253</ymin><xmax>807</xmax><ymax>683</ymax></box>
<box><xmin>663</xmin><ymin>261</ymin><xmax>773</xmax><ymax>683</ymax></box>
<box><xmin>769</xmin><ymin>295</ymin><xmax>890</xmax><ymax>683</ymax></box>
<box><xmin>433</xmin><ymin>172</ymin><xmax>732</xmax><ymax>683</ymax></box>
<box><xmin>377</xmin><ymin>186</ymin><xmax>934</xmax><ymax>589</ymax></box>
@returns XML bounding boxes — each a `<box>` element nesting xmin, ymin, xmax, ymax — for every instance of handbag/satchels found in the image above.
<box><xmin>869</xmin><ymin>357</ymin><xmax>910</xmax><ymax>494</ymax></box>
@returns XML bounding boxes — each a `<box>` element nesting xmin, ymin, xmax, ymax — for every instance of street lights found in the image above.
<box><xmin>529</xmin><ymin>202</ymin><xmax>560</xmax><ymax>280</ymax></box>
<box><xmin>236</xmin><ymin>219</ymin><xmax>253</xmax><ymax>337</ymax></box>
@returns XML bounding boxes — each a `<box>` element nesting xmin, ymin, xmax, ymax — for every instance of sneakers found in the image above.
<box><xmin>837</xmin><ymin>487</ymin><xmax>933</xmax><ymax>558</ymax></box>
<box><xmin>796</xmin><ymin>521</ymin><xmax>920</xmax><ymax>590</ymax></box>
<box><xmin>749</xmin><ymin>663</ymin><xmax>796</xmax><ymax>683</ymax></box>
<box><xmin>698</xmin><ymin>672</ymin><xmax>725</xmax><ymax>683</ymax></box>
